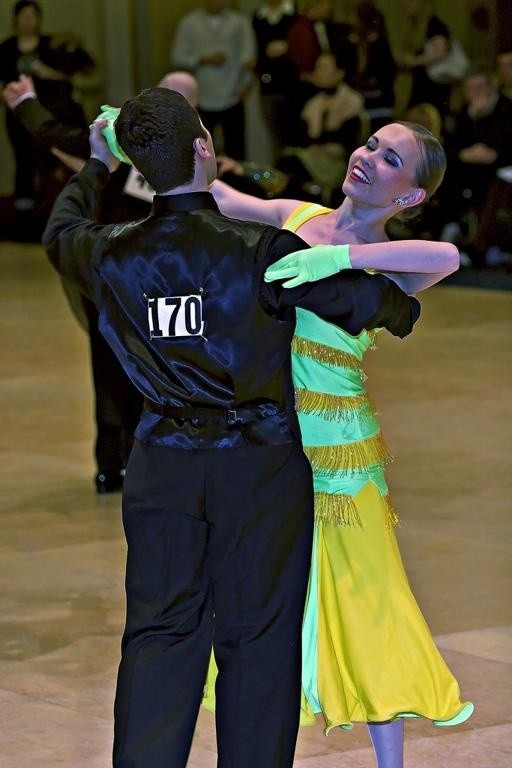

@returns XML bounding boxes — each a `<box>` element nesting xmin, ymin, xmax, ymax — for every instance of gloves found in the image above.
<box><xmin>264</xmin><ymin>243</ymin><xmax>354</xmax><ymax>289</ymax></box>
<box><xmin>99</xmin><ymin>105</ymin><xmax>138</xmax><ymax>168</ymax></box>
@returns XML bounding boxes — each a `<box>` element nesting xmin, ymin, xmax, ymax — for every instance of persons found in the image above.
<box><xmin>88</xmin><ymin>103</ymin><xmax>474</xmax><ymax>767</ymax></box>
<box><xmin>44</xmin><ymin>85</ymin><xmax>420</xmax><ymax>767</ymax></box>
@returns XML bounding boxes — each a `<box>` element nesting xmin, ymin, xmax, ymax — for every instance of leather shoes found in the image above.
<box><xmin>94</xmin><ymin>471</ymin><xmax>125</xmax><ymax>495</ymax></box>
